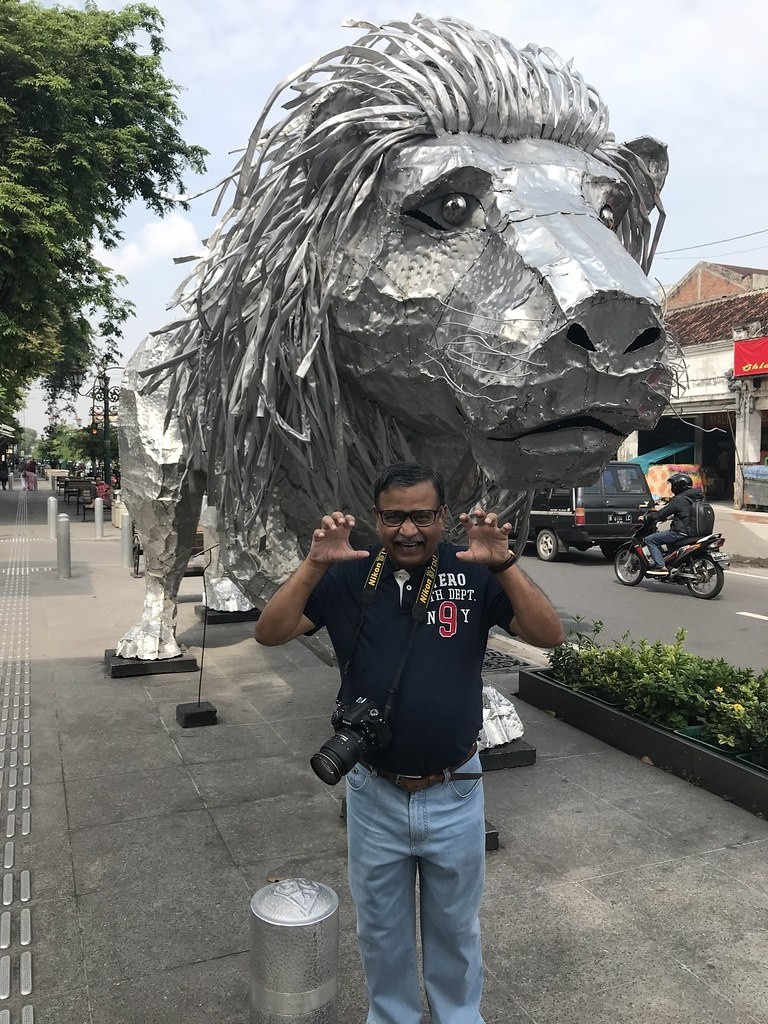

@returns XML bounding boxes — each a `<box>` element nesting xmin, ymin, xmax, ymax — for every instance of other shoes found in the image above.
<box><xmin>645</xmin><ymin>566</ymin><xmax>669</xmax><ymax>575</ymax></box>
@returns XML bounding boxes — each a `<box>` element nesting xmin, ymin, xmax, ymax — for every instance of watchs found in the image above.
<box><xmin>489</xmin><ymin>549</ymin><xmax>518</xmax><ymax>575</ymax></box>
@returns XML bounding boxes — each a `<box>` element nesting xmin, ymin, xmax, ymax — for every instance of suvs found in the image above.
<box><xmin>507</xmin><ymin>460</ymin><xmax>657</xmax><ymax>562</ymax></box>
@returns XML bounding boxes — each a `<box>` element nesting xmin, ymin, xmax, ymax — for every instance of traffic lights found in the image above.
<box><xmin>90</xmin><ymin>422</ymin><xmax>99</xmax><ymax>436</ymax></box>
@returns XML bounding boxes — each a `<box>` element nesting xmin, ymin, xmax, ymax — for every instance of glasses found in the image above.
<box><xmin>376</xmin><ymin>504</ymin><xmax>444</xmax><ymax>528</ymax></box>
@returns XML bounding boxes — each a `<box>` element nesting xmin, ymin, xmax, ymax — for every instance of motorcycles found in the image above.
<box><xmin>613</xmin><ymin>496</ymin><xmax>731</xmax><ymax>600</ymax></box>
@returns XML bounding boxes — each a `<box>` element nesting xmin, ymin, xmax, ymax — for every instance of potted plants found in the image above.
<box><xmin>537</xmin><ymin>614</ymin><xmax>768</xmax><ymax>776</ymax></box>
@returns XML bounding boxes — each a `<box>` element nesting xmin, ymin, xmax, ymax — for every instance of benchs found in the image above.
<box><xmin>56</xmin><ymin>476</ymin><xmax>117</xmax><ymax>522</ymax></box>
<box><xmin>129</xmin><ymin>520</ymin><xmax>205</xmax><ymax>578</ymax></box>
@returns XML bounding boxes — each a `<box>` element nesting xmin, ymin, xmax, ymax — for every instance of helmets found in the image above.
<box><xmin>667</xmin><ymin>473</ymin><xmax>693</xmax><ymax>496</ymax></box>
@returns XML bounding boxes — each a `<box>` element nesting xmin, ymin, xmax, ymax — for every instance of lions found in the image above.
<box><xmin>114</xmin><ymin>12</ymin><xmax>727</xmax><ymax>755</ymax></box>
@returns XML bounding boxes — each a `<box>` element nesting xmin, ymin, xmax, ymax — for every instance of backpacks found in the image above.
<box><xmin>678</xmin><ymin>489</ymin><xmax>716</xmax><ymax>537</ymax></box>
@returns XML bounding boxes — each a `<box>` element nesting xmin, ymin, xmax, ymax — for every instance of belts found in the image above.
<box><xmin>358</xmin><ymin>742</ymin><xmax>483</xmax><ymax>791</ymax></box>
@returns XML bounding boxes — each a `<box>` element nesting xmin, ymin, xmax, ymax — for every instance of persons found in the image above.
<box><xmin>638</xmin><ymin>473</ymin><xmax>707</xmax><ymax>575</ymax></box>
<box><xmin>0</xmin><ymin>458</ymin><xmax>121</xmax><ymax>512</ymax></box>
<box><xmin>253</xmin><ymin>462</ymin><xmax>565</xmax><ymax>1024</ymax></box>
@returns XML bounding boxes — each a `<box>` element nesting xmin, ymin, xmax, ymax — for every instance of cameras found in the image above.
<box><xmin>310</xmin><ymin>697</ymin><xmax>394</xmax><ymax>787</ymax></box>
